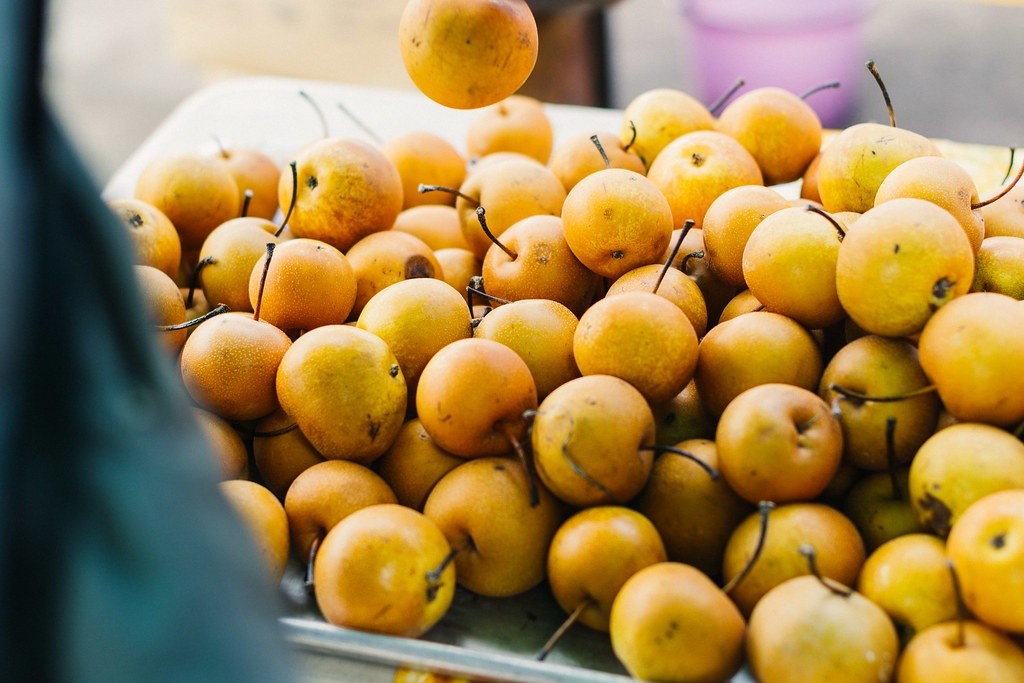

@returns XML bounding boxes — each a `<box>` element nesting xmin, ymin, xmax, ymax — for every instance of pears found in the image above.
<box><xmin>109</xmin><ymin>65</ymin><xmax>1023</xmax><ymax>681</ymax></box>
<box><xmin>401</xmin><ymin>0</ymin><xmax>538</xmax><ymax>109</ymax></box>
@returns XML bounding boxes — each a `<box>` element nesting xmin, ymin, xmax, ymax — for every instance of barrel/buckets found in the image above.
<box><xmin>682</xmin><ymin>0</ymin><xmax>881</xmax><ymax>129</ymax></box>
